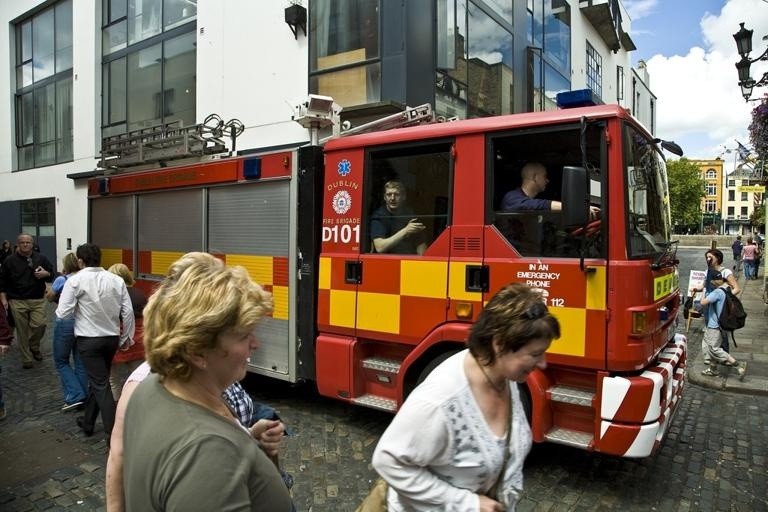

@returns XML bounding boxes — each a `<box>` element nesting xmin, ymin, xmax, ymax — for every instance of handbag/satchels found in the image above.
<box><xmin>355</xmin><ymin>480</ymin><xmax>387</xmax><ymax>512</ymax></box>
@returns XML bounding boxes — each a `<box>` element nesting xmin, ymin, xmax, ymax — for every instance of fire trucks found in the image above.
<box><xmin>74</xmin><ymin>85</ymin><xmax>694</xmax><ymax>466</ymax></box>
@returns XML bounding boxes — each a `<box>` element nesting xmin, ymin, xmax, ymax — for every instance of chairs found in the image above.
<box><xmin>685</xmin><ymin>288</ymin><xmax>706</xmax><ymax>334</ymax></box>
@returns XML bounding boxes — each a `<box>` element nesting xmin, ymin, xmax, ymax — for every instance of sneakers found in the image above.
<box><xmin>77</xmin><ymin>416</ymin><xmax>93</xmax><ymax>435</ymax></box>
<box><xmin>62</xmin><ymin>401</ymin><xmax>84</xmax><ymax>412</ymax></box>
<box><xmin>701</xmin><ymin>368</ymin><xmax>720</xmax><ymax>376</ymax></box>
<box><xmin>735</xmin><ymin>361</ymin><xmax>747</xmax><ymax>379</ymax></box>
<box><xmin>28</xmin><ymin>348</ymin><xmax>43</xmax><ymax>361</ymax></box>
<box><xmin>23</xmin><ymin>362</ymin><xmax>34</xmax><ymax>369</ymax></box>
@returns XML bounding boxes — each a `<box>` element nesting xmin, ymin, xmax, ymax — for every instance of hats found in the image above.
<box><xmin>705</xmin><ymin>270</ymin><xmax>722</xmax><ymax>280</ymax></box>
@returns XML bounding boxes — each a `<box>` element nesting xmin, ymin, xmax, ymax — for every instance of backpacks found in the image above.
<box><xmin>713</xmin><ymin>287</ymin><xmax>747</xmax><ymax>332</ymax></box>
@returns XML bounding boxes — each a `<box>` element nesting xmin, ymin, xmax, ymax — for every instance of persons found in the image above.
<box><xmin>499</xmin><ymin>160</ymin><xmax>602</xmax><ymax>256</ymax></box>
<box><xmin>104</xmin><ymin>358</ymin><xmax>255</xmax><ymax>511</ymax></box>
<box><xmin>122</xmin><ymin>249</ymin><xmax>294</xmax><ymax>512</ymax></box>
<box><xmin>369</xmin><ymin>180</ymin><xmax>431</xmax><ymax>257</ymax></box>
<box><xmin>0</xmin><ymin>231</ymin><xmax>149</xmax><ymax>449</ymax></box>
<box><xmin>689</xmin><ymin>230</ymin><xmax>763</xmax><ymax>382</ymax></box>
<box><xmin>371</xmin><ymin>282</ymin><xmax>562</xmax><ymax>511</ymax></box>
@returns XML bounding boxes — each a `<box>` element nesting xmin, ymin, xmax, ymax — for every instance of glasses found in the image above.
<box><xmin>519</xmin><ymin>302</ymin><xmax>549</xmax><ymax>321</ymax></box>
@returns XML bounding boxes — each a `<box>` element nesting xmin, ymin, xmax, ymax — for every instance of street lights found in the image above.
<box><xmin>732</xmin><ymin>20</ymin><xmax>768</xmax><ymax>103</ymax></box>
